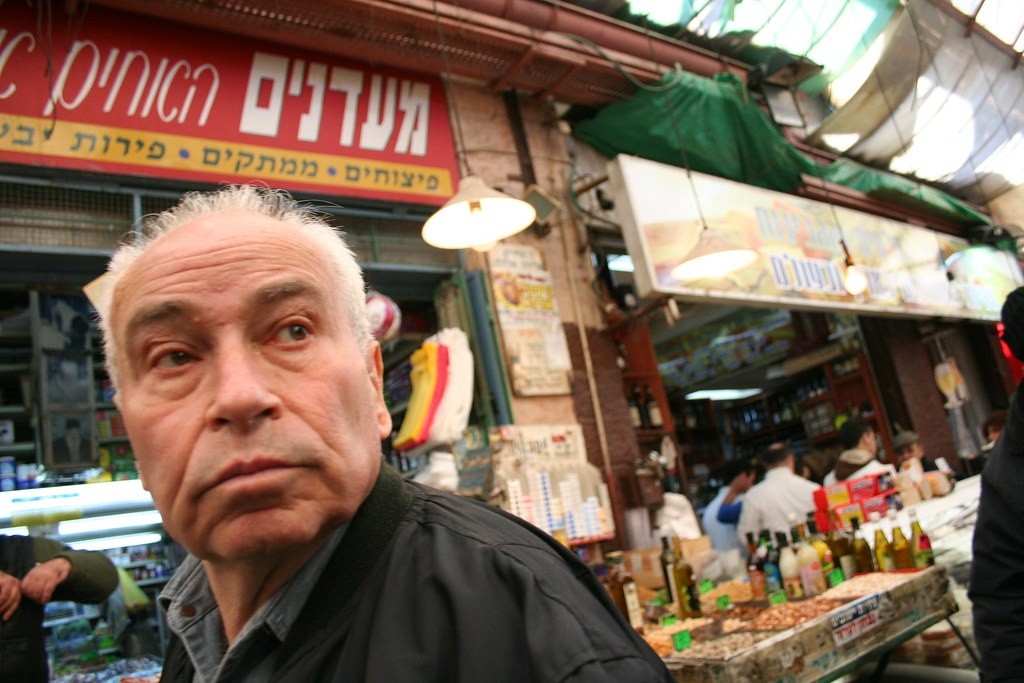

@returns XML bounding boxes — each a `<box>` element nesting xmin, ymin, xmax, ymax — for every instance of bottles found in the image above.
<box><xmin>657</xmin><ymin>535</ymin><xmax>703</xmax><ymax>619</ymax></box>
<box><xmin>624</xmin><ymin>380</ymin><xmax>662</xmax><ymax>427</ymax></box>
<box><xmin>748</xmin><ymin>513</ymin><xmax>950</xmax><ymax>605</ymax></box>
<box><xmin>95</xmin><ymin>619</ymin><xmax>116</xmax><ymax>648</ymax></box>
<box><xmin>604</xmin><ymin>551</ymin><xmax>644</xmax><ymax>635</ymax></box>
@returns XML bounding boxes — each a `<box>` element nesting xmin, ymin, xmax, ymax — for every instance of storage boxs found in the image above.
<box><xmin>812</xmin><ymin>472</ymin><xmax>906</xmax><ymax>531</ymax></box>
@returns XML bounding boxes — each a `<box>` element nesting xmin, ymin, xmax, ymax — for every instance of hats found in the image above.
<box><xmin>893</xmin><ymin>431</ymin><xmax>918</xmax><ymax>447</ymax></box>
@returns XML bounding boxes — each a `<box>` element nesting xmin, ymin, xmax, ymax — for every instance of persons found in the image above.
<box><xmin>0</xmin><ymin>535</ymin><xmax>118</xmax><ymax>683</ymax></box>
<box><xmin>54</xmin><ymin>419</ymin><xmax>90</xmax><ymax>463</ymax></box>
<box><xmin>823</xmin><ymin>418</ymin><xmax>893</xmax><ymax>487</ymax></box>
<box><xmin>892</xmin><ymin>431</ymin><xmax>939</xmax><ymax>473</ymax></box>
<box><xmin>969</xmin><ymin>285</ymin><xmax>1024</xmax><ymax>683</ymax></box>
<box><xmin>104</xmin><ymin>187</ymin><xmax>676</xmax><ymax>683</ymax></box>
<box><xmin>702</xmin><ymin>443</ymin><xmax>822</xmax><ymax>557</ymax></box>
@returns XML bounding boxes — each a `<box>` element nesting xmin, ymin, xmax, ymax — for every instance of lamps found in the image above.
<box><xmin>630</xmin><ymin>0</ymin><xmax>763</xmax><ymax>279</ymax></box>
<box><xmin>422</xmin><ymin>0</ymin><xmax>536</xmax><ymax>252</ymax></box>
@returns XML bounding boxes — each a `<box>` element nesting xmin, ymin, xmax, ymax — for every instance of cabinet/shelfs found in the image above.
<box><xmin>0</xmin><ymin>287</ymin><xmax>462</xmax><ymax>589</ymax></box>
<box><xmin>665</xmin><ymin>335</ymin><xmax>899</xmax><ymax>509</ymax></box>
